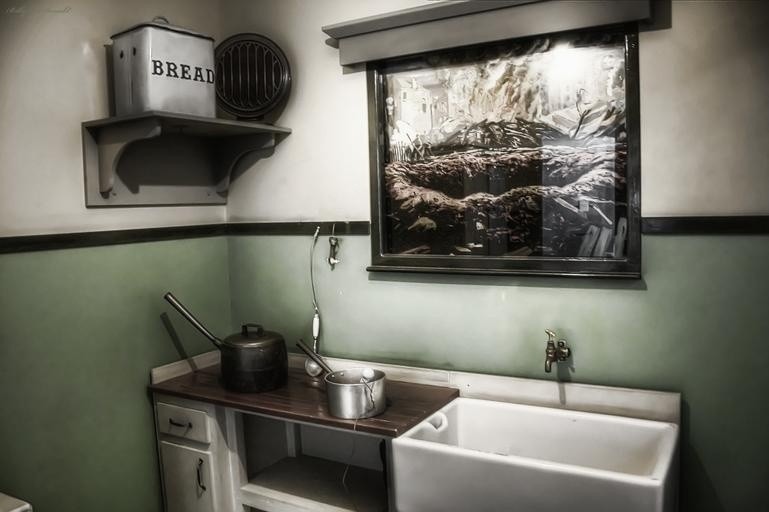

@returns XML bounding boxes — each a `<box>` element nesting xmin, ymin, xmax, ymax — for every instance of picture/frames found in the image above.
<box><xmin>359</xmin><ymin>21</ymin><xmax>645</xmax><ymax>284</ymax></box>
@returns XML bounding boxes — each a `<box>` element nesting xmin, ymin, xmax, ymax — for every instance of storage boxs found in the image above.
<box><xmin>104</xmin><ymin>15</ymin><xmax>221</xmax><ymax>122</ymax></box>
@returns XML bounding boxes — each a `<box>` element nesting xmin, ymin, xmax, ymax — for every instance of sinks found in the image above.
<box><xmin>391</xmin><ymin>397</ymin><xmax>680</xmax><ymax>512</ymax></box>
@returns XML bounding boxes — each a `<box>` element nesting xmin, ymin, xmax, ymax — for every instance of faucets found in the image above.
<box><xmin>544</xmin><ymin>329</ymin><xmax>570</xmax><ymax>373</ymax></box>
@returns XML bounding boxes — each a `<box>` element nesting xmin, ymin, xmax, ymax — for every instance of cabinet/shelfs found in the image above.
<box><xmin>144</xmin><ymin>388</ymin><xmax>243</xmax><ymax>512</ymax></box>
<box><xmin>226</xmin><ymin>404</ymin><xmax>397</xmax><ymax>512</ymax></box>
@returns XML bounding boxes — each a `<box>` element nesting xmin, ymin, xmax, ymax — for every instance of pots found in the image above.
<box><xmin>163</xmin><ymin>292</ymin><xmax>288</xmax><ymax>392</ymax></box>
<box><xmin>292</xmin><ymin>338</ymin><xmax>389</xmax><ymax>419</ymax></box>
